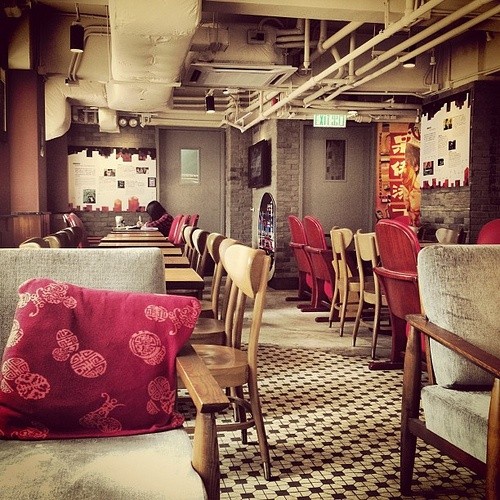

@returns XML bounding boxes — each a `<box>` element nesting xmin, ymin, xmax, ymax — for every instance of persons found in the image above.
<box><xmin>144</xmin><ymin>200</ymin><xmax>174</xmax><ymax>233</ymax></box>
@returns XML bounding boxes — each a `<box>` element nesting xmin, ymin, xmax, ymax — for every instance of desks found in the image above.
<box><xmin>97</xmin><ymin>242</ymin><xmax>176</xmax><ymax>248</ymax></box>
<box><xmin>106</xmin><ymin>231</ymin><xmax>164</xmax><ymax>236</ymax></box>
<box><xmin>163</xmin><ymin>267</ymin><xmax>206</xmax><ymax>298</ymax></box>
<box><xmin>165</xmin><ymin>256</ymin><xmax>190</xmax><ymax>268</ymax></box>
<box><xmin>101</xmin><ymin>236</ymin><xmax>169</xmax><ymax>242</ymax></box>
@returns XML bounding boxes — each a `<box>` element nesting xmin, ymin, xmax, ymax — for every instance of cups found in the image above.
<box><xmin>115</xmin><ymin>215</ymin><xmax>124</xmax><ymax>227</ymax></box>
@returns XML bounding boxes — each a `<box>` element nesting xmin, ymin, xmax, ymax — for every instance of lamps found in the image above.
<box><xmin>486</xmin><ymin>32</ymin><xmax>493</xmax><ymax>41</ymax></box>
<box><xmin>430</xmin><ymin>55</ymin><xmax>436</xmax><ymax>65</ymax></box>
<box><xmin>402</xmin><ymin>29</ymin><xmax>416</xmax><ymax>68</ymax></box>
<box><xmin>70</xmin><ymin>17</ymin><xmax>85</xmax><ymax>54</ymax></box>
<box><xmin>204</xmin><ymin>92</ymin><xmax>216</xmax><ymax>113</ymax></box>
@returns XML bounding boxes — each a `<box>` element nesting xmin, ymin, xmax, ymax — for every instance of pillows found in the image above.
<box><xmin>0</xmin><ymin>278</ymin><xmax>203</xmax><ymax>442</ymax></box>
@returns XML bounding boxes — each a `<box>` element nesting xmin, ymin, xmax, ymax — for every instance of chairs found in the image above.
<box><xmin>1</xmin><ymin>212</ymin><xmax>272</xmax><ymax>500</ymax></box>
<box><xmin>285</xmin><ymin>212</ymin><xmax>500</xmax><ymax>500</ymax></box>
